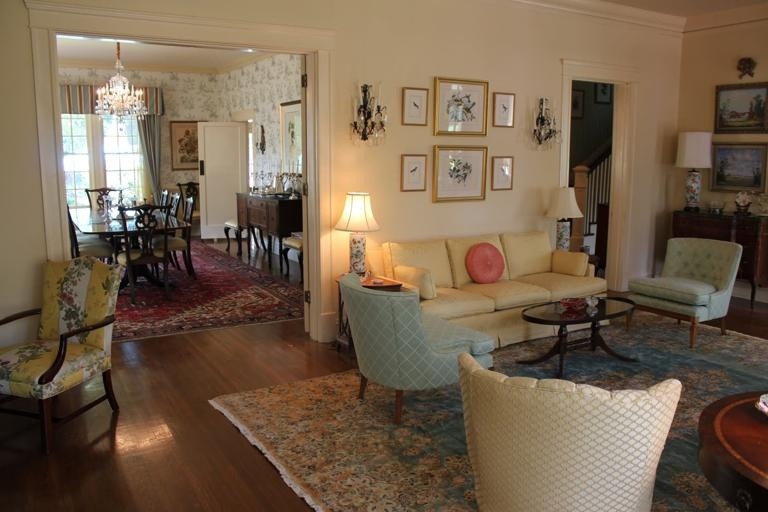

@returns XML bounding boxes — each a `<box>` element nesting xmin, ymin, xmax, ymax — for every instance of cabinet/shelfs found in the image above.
<box><xmin>235</xmin><ymin>191</ymin><xmax>302</xmax><ymax>272</ymax></box>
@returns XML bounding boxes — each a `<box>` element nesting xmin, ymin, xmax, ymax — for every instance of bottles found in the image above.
<box><xmin>253</xmin><ymin>169</ymin><xmax>302</xmax><ymax>194</ymax></box>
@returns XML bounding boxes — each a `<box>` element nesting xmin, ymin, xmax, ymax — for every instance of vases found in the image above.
<box><xmin>735</xmin><ymin>203</ymin><xmax>750</xmax><ymax>213</ymax></box>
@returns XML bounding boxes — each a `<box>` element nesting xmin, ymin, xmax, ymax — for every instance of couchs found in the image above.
<box><xmin>338</xmin><ymin>230</ymin><xmax>608</xmax><ymax>426</ymax></box>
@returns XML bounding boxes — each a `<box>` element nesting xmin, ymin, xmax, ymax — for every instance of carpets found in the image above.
<box><xmin>97</xmin><ymin>240</ymin><xmax>304</xmax><ymax>344</ymax></box>
<box><xmin>207</xmin><ymin>311</ymin><xmax>767</xmax><ymax>511</ymax></box>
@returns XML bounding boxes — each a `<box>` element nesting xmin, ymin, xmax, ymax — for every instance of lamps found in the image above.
<box><xmin>332</xmin><ymin>189</ymin><xmax>380</xmax><ymax>280</ymax></box>
<box><xmin>349</xmin><ymin>81</ymin><xmax>390</xmax><ymax>146</ymax></box>
<box><xmin>531</xmin><ymin>97</ymin><xmax>564</xmax><ymax>148</ymax></box>
<box><xmin>673</xmin><ymin>130</ymin><xmax>713</xmax><ymax>212</ymax></box>
<box><xmin>94</xmin><ymin>42</ymin><xmax>148</xmax><ymax>132</ymax></box>
<box><xmin>543</xmin><ymin>186</ymin><xmax>584</xmax><ymax>252</ymax></box>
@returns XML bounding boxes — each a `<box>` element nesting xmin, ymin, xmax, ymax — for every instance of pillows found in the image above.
<box><xmin>393</xmin><ymin>264</ymin><xmax>437</xmax><ymax>299</ymax></box>
<box><xmin>464</xmin><ymin>242</ymin><xmax>506</xmax><ymax>284</ymax></box>
<box><xmin>551</xmin><ymin>248</ymin><xmax>589</xmax><ymax>277</ymax></box>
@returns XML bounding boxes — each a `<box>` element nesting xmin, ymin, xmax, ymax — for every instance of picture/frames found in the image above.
<box><xmin>277</xmin><ymin>99</ymin><xmax>302</xmax><ymax>175</ymax></box>
<box><xmin>401</xmin><ymin>87</ymin><xmax>429</xmax><ymax>127</ymax></box>
<box><xmin>707</xmin><ymin>141</ymin><xmax>768</xmax><ymax>195</ymax></box>
<box><xmin>594</xmin><ymin>83</ymin><xmax>611</xmax><ymax>104</ymax></box>
<box><xmin>493</xmin><ymin>90</ymin><xmax>516</xmax><ymax>129</ymax></box>
<box><xmin>432</xmin><ymin>143</ymin><xmax>488</xmax><ymax>203</ymax></box>
<box><xmin>571</xmin><ymin>89</ymin><xmax>585</xmax><ymax>119</ymax></box>
<box><xmin>399</xmin><ymin>153</ymin><xmax>428</xmax><ymax>192</ymax></box>
<box><xmin>711</xmin><ymin>81</ymin><xmax>768</xmax><ymax>135</ymax></box>
<box><xmin>433</xmin><ymin>75</ymin><xmax>489</xmax><ymax>137</ymax></box>
<box><xmin>491</xmin><ymin>156</ymin><xmax>514</xmax><ymax>192</ymax></box>
<box><xmin>168</xmin><ymin>119</ymin><xmax>208</xmax><ymax>171</ymax></box>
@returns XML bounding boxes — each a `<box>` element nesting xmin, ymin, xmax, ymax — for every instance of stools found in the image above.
<box><xmin>281</xmin><ymin>236</ymin><xmax>303</xmax><ymax>286</ymax></box>
<box><xmin>223</xmin><ymin>219</ymin><xmax>260</xmax><ymax>256</ymax></box>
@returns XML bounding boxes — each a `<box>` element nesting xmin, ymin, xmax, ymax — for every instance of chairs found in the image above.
<box><xmin>0</xmin><ymin>254</ymin><xmax>126</xmax><ymax>456</ymax></box>
<box><xmin>458</xmin><ymin>348</ymin><xmax>683</xmax><ymax>512</ymax></box>
<box><xmin>67</xmin><ymin>187</ymin><xmax>197</xmax><ymax>303</ymax></box>
<box><xmin>624</xmin><ymin>236</ymin><xmax>743</xmax><ymax>349</ymax></box>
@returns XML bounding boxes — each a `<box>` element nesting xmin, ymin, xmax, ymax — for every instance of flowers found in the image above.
<box><xmin>734</xmin><ymin>190</ymin><xmax>753</xmax><ymax>206</ymax></box>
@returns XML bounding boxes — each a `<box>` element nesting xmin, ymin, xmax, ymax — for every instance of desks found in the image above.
<box><xmin>671</xmin><ymin>207</ymin><xmax>768</xmax><ymax>310</ymax></box>
<box><xmin>327</xmin><ymin>271</ymin><xmax>402</xmax><ymax>358</ymax></box>
<box><xmin>514</xmin><ymin>296</ymin><xmax>638</xmax><ymax>380</ymax></box>
<box><xmin>695</xmin><ymin>389</ymin><xmax>768</xmax><ymax>511</ymax></box>
